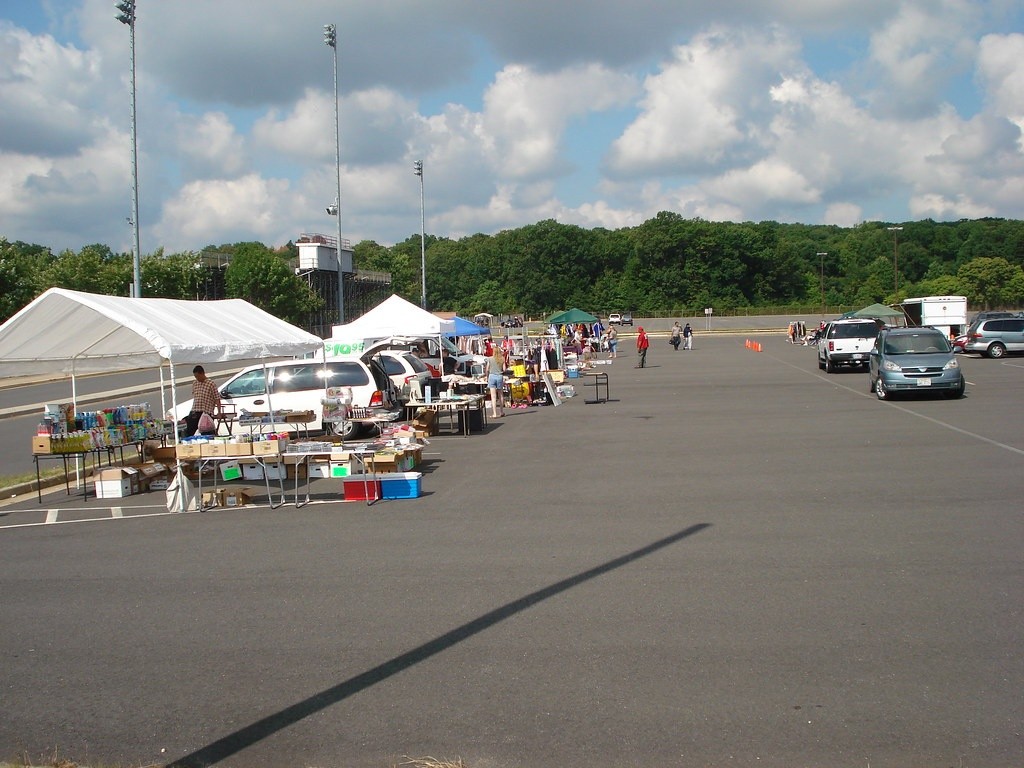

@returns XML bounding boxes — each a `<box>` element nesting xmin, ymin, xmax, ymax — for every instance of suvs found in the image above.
<box><xmin>816</xmin><ymin>317</ymin><xmax>881</xmax><ymax>374</ymax></box>
<box><xmin>869</xmin><ymin>326</ymin><xmax>966</xmax><ymax>400</ymax></box>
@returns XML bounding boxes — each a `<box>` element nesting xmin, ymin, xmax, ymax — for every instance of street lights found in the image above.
<box><xmin>113</xmin><ymin>1</ymin><xmax>141</xmax><ymax>298</ymax></box>
<box><xmin>324</xmin><ymin>23</ymin><xmax>344</xmax><ymax>324</ymax></box>
<box><xmin>887</xmin><ymin>227</ymin><xmax>903</xmax><ymax>303</ymax></box>
<box><xmin>816</xmin><ymin>252</ymin><xmax>828</xmax><ymax>318</ymax></box>
<box><xmin>414</xmin><ymin>160</ymin><xmax>425</xmax><ymax>311</ymax></box>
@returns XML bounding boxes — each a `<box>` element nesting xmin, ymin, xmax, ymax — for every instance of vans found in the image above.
<box><xmin>964</xmin><ymin>318</ymin><xmax>1024</xmax><ymax>359</ymax></box>
<box><xmin>978</xmin><ymin>312</ymin><xmax>1014</xmax><ymax>320</ymax></box>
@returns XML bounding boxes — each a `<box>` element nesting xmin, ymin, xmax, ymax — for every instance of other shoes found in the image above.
<box><xmin>490</xmin><ymin>415</ymin><xmax>496</xmax><ymax>419</ymax></box>
<box><xmin>501</xmin><ymin>413</ymin><xmax>506</xmax><ymax>416</ymax></box>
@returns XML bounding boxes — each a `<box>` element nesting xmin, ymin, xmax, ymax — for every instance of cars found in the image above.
<box><xmin>504</xmin><ymin>318</ymin><xmax>518</xmax><ymax>328</ymax></box>
<box><xmin>620</xmin><ymin>315</ymin><xmax>633</xmax><ymax>327</ymax></box>
<box><xmin>609</xmin><ymin>314</ymin><xmax>621</xmax><ymax>326</ymax></box>
<box><xmin>952</xmin><ymin>334</ymin><xmax>971</xmax><ymax>353</ymax></box>
<box><xmin>352</xmin><ymin>352</ymin><xmax>434</xmax><ymax>422</ymax></box>
<box><xmin>969</xmin><ymin>313</ymin><xmax>979</xmax><ymax>326</ymax></box>
<box><xmin>388</xmin><ymin>335</ymin><xmax>489</xmax><ymax>380</ymax></box>
<box><xmin>165</xmin><ymin>336</ymin><xmax>426</xmax><ymax>442</ymax></box>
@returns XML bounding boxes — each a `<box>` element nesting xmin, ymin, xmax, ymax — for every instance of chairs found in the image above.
<box><xmin>213</xmin><ymin>404</ymin><xmax>238</xmax><ymax>440</ymax></box>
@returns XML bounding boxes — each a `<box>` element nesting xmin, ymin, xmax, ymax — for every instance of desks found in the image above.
<box><xmin>405</xmin><ymin>401</ymin><xmax>470</xmax><ymax>437</ymax></box>
<box><xmin>415</xmin><ymin>394</ymin><xmax>487</xmax><ymax>429</ymax></box>
<box><xmin>31</xmin><ymin>431</ymin><xmax>182</xmax><ymax>503</ymax></box>
<box><xmin>183</xmin><ymin>450</ymin><xmax>384</xmax><ymax>513</ymax></box>
<box><xmin>239</xmin><ymin>422</ymin><xmax>309</xmax><ymax>439</ymax></box>
<box><xmin>453</xmin><ymin>378</ymin><xmax>523</xmax><ymax>408</ymax></box>
<box><xmin>323</xmin><ymin>416</ymin><xmax>399</xmax><ymax>443</ymax></box>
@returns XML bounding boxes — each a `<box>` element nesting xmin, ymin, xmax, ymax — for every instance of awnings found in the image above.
<box><xmin>332</xmin><ymin>294</ymin><xmax>455</xmax><ymax>409</ymax></box>
<box><xmin>441</xmin><ymin>317</ymin><xmax>490</xmax><ymax>355</ymax></box>
<box><xmin>544</xmin><ymin>308</ymin><xmax>600</xmax><ymax>341</ymax></box>
<box><xmin>838</xmin><ymin>303</ymin><xmax>904</xmax><ymax>330</ymax></box>
<box><xmin>0</xmin><ymin>287</ymin><xmax>327</xmax><ymax>512</ymax></box>
<box><xmin>475</xmin><ymin>313</ymin><xmax>493</xmax><ymax>326</ymax></box>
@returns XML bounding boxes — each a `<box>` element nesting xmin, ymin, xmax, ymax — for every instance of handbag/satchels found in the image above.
<box><xmin>669</xmin><ymin>340</ymin><xmax>675</xmax><ymax>345</ymax></box>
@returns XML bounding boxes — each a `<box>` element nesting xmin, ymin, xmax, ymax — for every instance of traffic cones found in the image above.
<box><xmin>746</xmin><ymin>339</ymin><xmax>763</xmax><ymax>352</ymax></box>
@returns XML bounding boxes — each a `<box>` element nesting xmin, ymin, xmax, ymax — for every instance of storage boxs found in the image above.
<box><xmin>92</xmin><ymin>409</ymin><xmax>437</xmax><ymax>507</ymax></box>
<box><xmin>412</xmin><ymin>410</ymin><xmax>439</xmax><ymax>437</ymax></box>
<box><xmin>32</xmin><ymin>436</ymin><xmax>56</xmax><ymax>454</ymax></box>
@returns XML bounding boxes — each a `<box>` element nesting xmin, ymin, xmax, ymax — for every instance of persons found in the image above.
<box><xmin>439</xmin><ymin>349</ymin><xmax>460</xmax><ymax>375</ymax></box>
<box><xmin>671</xmin><ymin>322</ymin><xmax>682</xmax><ymax>351</ymax></box>
<box><xmin>485</xmin><ymin>334</ymin><xmax>528</xmax><ymax>359</ymax></box>
<box><xmin>188</xmin><ymin>365</ymin><xmax>221</xmax><ymax>436</ymax></box>
<box><xmin>683</xmin><ymin>323</ymin><xmax>692</xmax><ymax>351</ymax></box>
<box><xmin>634</xmin><ymin>327</ymin><xmax>649</xmax><ymax>368</ymax></box>
<box><xmin>241</xmin><ymin>384</ymin><xmax>267</xmax><ymax>393</ymax></box>
<box><xmin>532</xmin><ymin>338</ymin><xmax>541</xmax><ymax>381</ymax></box>
<box><xmin>801</xmin><ymin>321</ymin><xmax>827</xmax><ymax>346</ymax></box>
<box><xmin>449</xmin><ymin>336</ymin><xmax>459</xmax><ymax>345</ymax></box>
<box><xmin>574</xmin><ymin>327</ymin><xmax>583</xmax><ymax>354</ymax></box>
<box><xmin>486</xmin><ymin>347</ymin><xmax>506</xmax><ymax>419</ymax></box>
<box><xmin>412</xmin><ymin>348</ymin><xmax>420</xmax><ymax>357</ymax></box>
<box><xmin>601</xmin><ymin>326</ymin><xmax>617</xmax><ymax>359</ymax></box>
